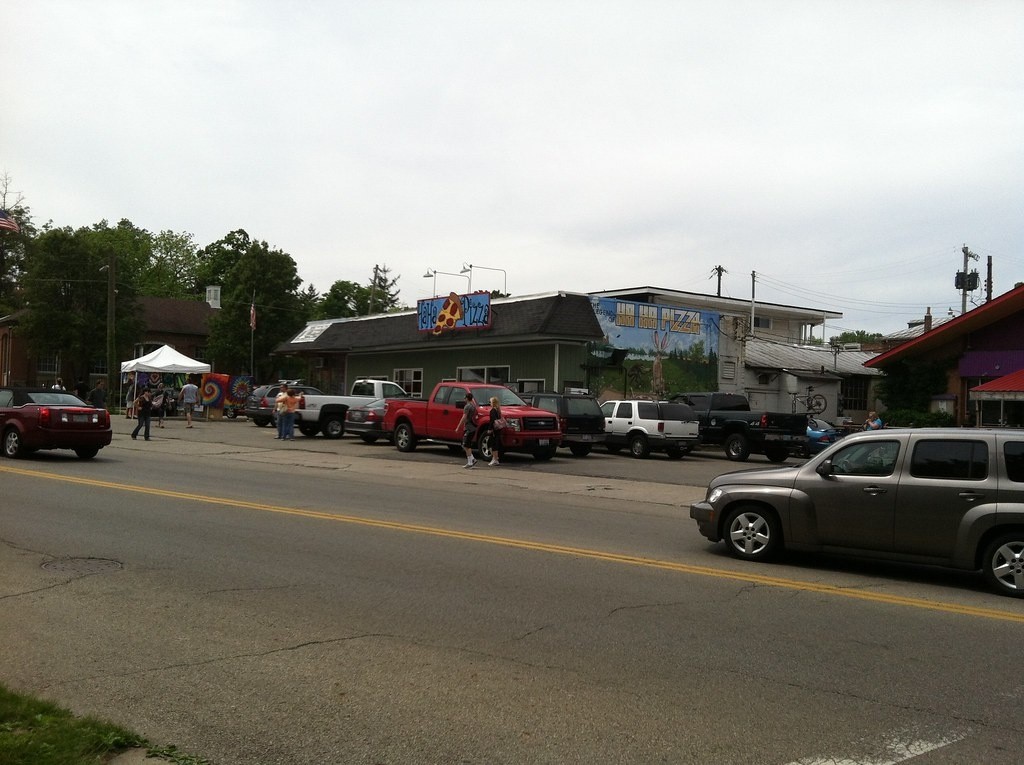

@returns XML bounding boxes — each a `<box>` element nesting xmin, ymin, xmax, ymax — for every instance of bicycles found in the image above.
<box><xmin>789</xmin><ymin>386</ymin><xmax>827</xmax><ymax>414</ymax></box>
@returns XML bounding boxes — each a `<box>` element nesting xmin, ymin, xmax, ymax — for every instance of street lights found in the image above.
<box><xmin>423</xmin><ymin>262</ymin><xmax>507</xmax><ymax>298</ymax></box>
<box><xmin>99</xmin><ymin>265</ymin><xmax>116</xmax><ymax>414</ymax></box>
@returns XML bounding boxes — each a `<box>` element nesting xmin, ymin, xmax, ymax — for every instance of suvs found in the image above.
<box><xmin>689</xmin><ymin>427</ymin><xmax>1024</xmax><ymax>599</ymax></box>
<box><xmin>600</xmin><ymin>399</ymin><xmax>703</xmax><ymax>459</ymax></box>
<box><xmin>516</xmin><ymin>391</ymin><xmax>613</xmax><ymax>457</ymax></box>
<box><xmin>224</xmin><ymin>380</ymin><xmax>326</xmax><ymax>428</ymax></box>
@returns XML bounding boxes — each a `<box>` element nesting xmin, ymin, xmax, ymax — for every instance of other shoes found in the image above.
<box><xmin>472</xmin><ymin>458</ymin><xmax>478</xmax><ymax>465</ymax></box>
<box><xmin>159</xmin><ymin>421</ymin><xmax>164</xmax><ymax>428</ymax></box>
<box><xmin>185</xmin><ymin>425</ymin><xmax>192</xmax><ymax>428</ymax></box>
<box><xmin>280</xmin><ymin>438</ymin><xmax>284</xmax><ymax>441</ymax></box>
<box><xmin>274</xmin><ymin>435</ymin><xmax>280</xmax><ymax>439</ymax></box>
<box><xmin>126</xmin><ymin>415</ymin><xmax>131</xmax><ymax>419</ymax></box>
<box><xmin>492</xmin><ymin>456</ymin><xmax>499</xmax><ymax>460</ymax></box>
<box><xmin>290</xmin><ymin>438</ymin><xmax>295</xmax><ymax>441</ymax></box>
<box><xmin>462</xmin><ymin>463</ymin><xmax>473</xmax><ymax>468</ymax></box>
<box><xmin>131</xmin><ymin>434</ymin><xmax>136</xmax><ymax>440</ymax></box>
<box><xmin>133</xmin><ymin>416</ymin><xmax>138</xmax><ymax>419</ymax></box>
<box><xmin>488</xmin><ymin>460</ymin><xmax>499</xmax><ymax>467</ymax></box>
<box><xmin>285</xmin><ymin>435</ymin><xmax>290</xmax><ymax>439</ymax></box>
<box><xmin>144</xmin><ymin>436</ymin><xmax>151</xmax><ymax>441</ymax></box>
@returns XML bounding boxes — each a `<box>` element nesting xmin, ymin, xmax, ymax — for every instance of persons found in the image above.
<box><xmin>131</xmin><ymin>388</ymin><xmax>151</xmax><ymax>440</ymax></box>
<box><xmin>126</xmin><ymin>379</ymin><xmax>139</xmax><ymax>419</ymax></box>
<box><xmin>486</xmin><ymin>397</ymin><xmax>500</xmax><ymax>467</ymax></box>
<box><xmin>272</xmin><ymin>384</ymin><xmax>304</xmax><ymax>441</ymax></box>
<box><xmin>456</xmin><ymin>393</ymin><xmax>478</xmax><ymax>468</ymax></box>
<box><xmin>52</xmin><ymin>377</ymin><xmax>66</xmax><ymax>390</ymax></box>
<box><xmin>178</xmin><ymin>378</ymin><xmax>200</xmax><ymax>428</ymax></box>
<box><xmin>86</xmin><ymin>379</ymin><xmax>106</xmax><ymax>408</ymax></box>
<box><xmin>151</xmin><ymin>383</ymin><xmax>168</xmax><ymax>429</ymax></box>
<box><xmin>863</xmin><ymin>412</ymin><xmax>882</xmax><ymax>430</ymax></box>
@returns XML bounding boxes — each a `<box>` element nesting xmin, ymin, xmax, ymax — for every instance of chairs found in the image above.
<box><xmin>454</xmin><ymin>392</ymin><xmax>464</xmax><ymax>401</ymax></box>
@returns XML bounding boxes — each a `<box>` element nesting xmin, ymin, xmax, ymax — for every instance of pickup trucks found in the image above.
<box><xmin>382</xmin><ymin>383</ymin><xmax>562</xmax><ymax>462</ymax></box>
<box><xmin>670</xmin><ymin>392</ymin><xmax>811</xmax><ymax>462</ymax></box>
<box><xmin>293</xmin><ymin>379</ymin><xmax>413</xmax><ymax>446</ymax></box>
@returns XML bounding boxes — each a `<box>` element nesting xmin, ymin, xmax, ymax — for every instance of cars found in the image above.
<box><xmin>0</xmin><ymin>387</ymin><xmax>113</xmax><ymax>459</ymax></box>
<box><xmin>806</xmin><ymin>419</ymin><xmax>844</xmax><ymax>454</ymax></box>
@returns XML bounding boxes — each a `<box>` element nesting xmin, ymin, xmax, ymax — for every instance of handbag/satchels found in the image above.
<box><xmin>152</xmin><ymin>394</ymin><xmax>164</xmax><ymax>408</ymax></box>
<box><xmin>493</xmin><ymin>417</ymin><xmax>507</xmax><ymax>430</ymax></box>
<box><xmin>279</xmin><ymin>396</ymin><xmax>288</xmax><ymax>416</ymax></box>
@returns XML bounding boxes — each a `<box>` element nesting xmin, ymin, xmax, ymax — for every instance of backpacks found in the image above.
<box><xmin>470</xmin><ymin>403</ymin><xmax>479</xmax><ymax>426</ymax></box>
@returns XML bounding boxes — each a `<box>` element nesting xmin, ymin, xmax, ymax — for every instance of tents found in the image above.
<box><xmin>121</xmin><ymin>344</ymin><xmax>211</xmax><ymax>422</ymax></box>
